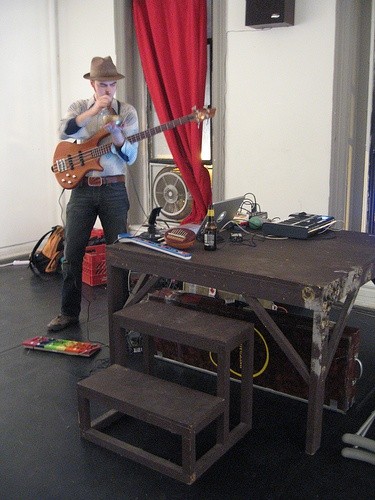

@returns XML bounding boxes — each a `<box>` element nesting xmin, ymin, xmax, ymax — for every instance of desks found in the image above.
<box><xmin>106</xmin><ymin>222</ymin><xmax>375</xmax><ymax>456</ymax></box>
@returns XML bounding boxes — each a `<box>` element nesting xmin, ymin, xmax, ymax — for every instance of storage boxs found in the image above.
<box><xmin>82</xmin><ymin>229</ymin><xmax>108</xmax><ymax>286</ymax></box>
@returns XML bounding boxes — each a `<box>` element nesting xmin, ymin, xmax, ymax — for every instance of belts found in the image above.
<box><xmin>83</xmin><ymin>175</ymin><xmax>126</xmax><ymax>187</ymax></box>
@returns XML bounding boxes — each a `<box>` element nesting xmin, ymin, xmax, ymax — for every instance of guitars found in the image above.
<box><xmin>50</xmin><ymin>104</ymin><xmax>219</xmax><ymax>191</ymax></box>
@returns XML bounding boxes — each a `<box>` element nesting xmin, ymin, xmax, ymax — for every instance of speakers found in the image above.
<box><xmin>245</xmin><ymin>0</ymin><xmax>295</xmax><ymax>30</ymax></box>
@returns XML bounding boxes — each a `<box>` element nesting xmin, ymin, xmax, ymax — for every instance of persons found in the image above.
<box><xmin>48</xmin><ymin>56</ymin><xmax>139</xmax><ymax>332</ymax></box>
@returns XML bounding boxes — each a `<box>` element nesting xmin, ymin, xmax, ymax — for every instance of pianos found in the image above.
<box><xmin>261</xmin><ymin>212</ymin><xmax>336</xmax><ymax>240</ymax></box>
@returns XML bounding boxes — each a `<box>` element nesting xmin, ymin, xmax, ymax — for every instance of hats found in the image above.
<box><xmin>82</xmin><ymin>55</ymin><xmax>126</xmax><ymax>82</ymax></box>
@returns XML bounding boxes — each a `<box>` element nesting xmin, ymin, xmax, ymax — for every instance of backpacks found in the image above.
<box><xmin>26</xmin><ymin>225</ymin><xmax>65</xmax><ymax>276</ymax></box>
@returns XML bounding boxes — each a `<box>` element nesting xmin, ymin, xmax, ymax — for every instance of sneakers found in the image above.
<box><xmin>46</xmin><ymin>311</ymin><xmax>81</xmax><ymax>332</ymax></box>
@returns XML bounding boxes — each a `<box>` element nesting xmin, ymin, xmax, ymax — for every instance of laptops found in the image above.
<box><xmin>177</xmin><ymin>196</ymin><xmax>245</xmax><ymax>241</ymax></box>
